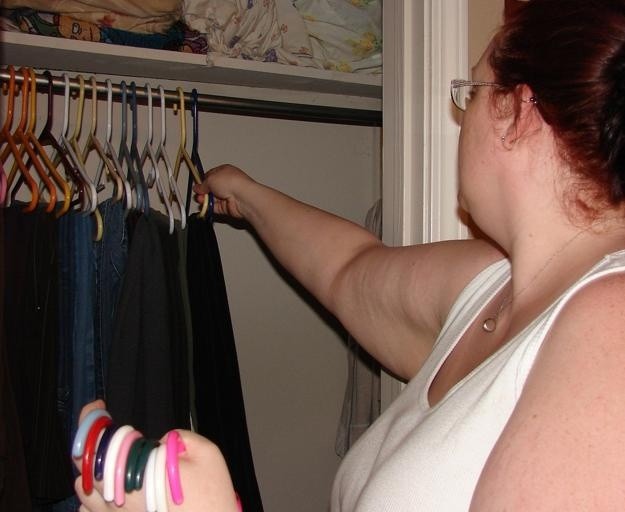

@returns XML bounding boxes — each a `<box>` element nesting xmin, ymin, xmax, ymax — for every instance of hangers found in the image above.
<box><xmin>72</xmin><ymin>409</ymin><xmax>242</xmax><ymax>512</ymax></box>
<box><xmin>0</xmin><ymin>64</ymin><xmax>214</xmax><ymax>242</ymax></box>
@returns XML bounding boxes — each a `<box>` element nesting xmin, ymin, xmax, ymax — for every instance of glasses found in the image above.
<box><xmin>451</xmin><ymin>79</ymin><xmax>499</xmax><ymax>112</ymax></box>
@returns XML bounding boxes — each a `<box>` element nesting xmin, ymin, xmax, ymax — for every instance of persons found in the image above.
<box><xmin>68</xmin><ymin>0</ymin><xmax>624</xmax><ymax>512</ymax></box>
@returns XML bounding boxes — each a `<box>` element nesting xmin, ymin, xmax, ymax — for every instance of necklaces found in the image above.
<box><xmin>480</xmin><ymin>211</ymin><xmax>625</xmax><ymax>333</ymax></box>
